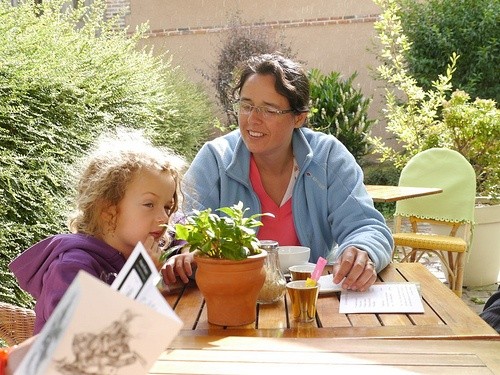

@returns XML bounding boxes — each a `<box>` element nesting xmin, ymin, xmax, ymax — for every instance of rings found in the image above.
<box><xmin>366</xmin><ymin>261</ymin><xmax>376</xmax><ymax>268</ymax></box>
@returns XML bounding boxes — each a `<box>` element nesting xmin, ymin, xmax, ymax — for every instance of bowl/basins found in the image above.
<box><xmin>279</xmin><ymin>246</ymin><xmax>310</xmax><ymax>275</ymax></box>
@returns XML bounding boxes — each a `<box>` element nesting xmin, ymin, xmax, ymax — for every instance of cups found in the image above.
<box><xmin>286</xmin><ymin>280</ymin><xmax>321</xmax><ymax>322</ymax></box>
<box><xmin>288</xmin><ymin>265</ymin><xmax>315</xmax><ymax>281</ymax></box>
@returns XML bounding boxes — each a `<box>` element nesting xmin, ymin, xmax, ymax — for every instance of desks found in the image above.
<box><xmin>363</xmin><ymin>184</ymin><xmax>444</xmax><ymax>202</ymax></box>
<box><xmin>144</xmin><ymin>337</ymin><xmax>500</xmax><ymax>375</ymax></box>
<box><xmin>147</xmin><ymin>262</ymin><xmax>500</xmax><ymax>339</ymax></box>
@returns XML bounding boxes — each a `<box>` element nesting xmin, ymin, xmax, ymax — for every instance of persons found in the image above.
<box><xmin>162</xmin><ymin>51</ymin><xmax>395</xmax><ymax>293</ymax></box>
<box><xmin>8</xmin><ymin>127</ymin><xmax>190</xmax><ymax>337</ymax></box>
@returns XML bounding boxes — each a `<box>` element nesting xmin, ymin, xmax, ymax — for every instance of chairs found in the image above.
<box><xmin>0</xmin><ymin>302</ymin><xmax>37</xmax><ymax>349</ymax></box>
<box><xmin>391</xmin><ymin>147</ymin><xmax>476</xmax><ymax>298</ymax></box>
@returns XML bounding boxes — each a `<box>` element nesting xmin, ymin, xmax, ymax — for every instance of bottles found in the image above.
<box><xmin>256</xmin><ymin>241</ymin><xmax>285</xmax><ymax>304</ymax></box>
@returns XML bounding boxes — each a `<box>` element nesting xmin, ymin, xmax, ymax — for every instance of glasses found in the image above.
<box><xmin>233</xmin><ymin>101</ymin><xmax>296</xmax><ymax>119</ymax></box>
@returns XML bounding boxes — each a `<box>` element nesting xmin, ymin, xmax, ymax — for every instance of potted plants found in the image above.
<box><xmin>158</xmin><ymin>201</ymin><xmax>276</xmax><ymax>327</ymax></box>
